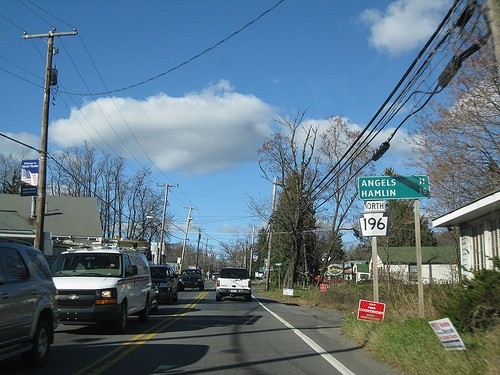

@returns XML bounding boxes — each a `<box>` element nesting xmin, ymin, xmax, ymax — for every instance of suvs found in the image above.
<box><xmin>0</xmin><ymin>237</ymin><xmax>60</xmax><ymax>369</ymax></box>
<box><xmin>49</xmin><ymin>236</ymin><xmax>153</xmax><ymax>332</ymax></box>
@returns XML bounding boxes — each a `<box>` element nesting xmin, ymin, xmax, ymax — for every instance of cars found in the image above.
<box><xmin>215</xmin><ymin>267</ymin><xmax>255</xmax><ymax>301</ymax></box>
<box><xmin>177</xmin><ymin>269</ymin><xmax>205</xmax><ymax>292</ymax></box>
<box><xmin>212</xmin><ymin>273</ymin><xmax>220</xmax><ymax>281</ymax></box>
<box><xmin>149</xmin><ymin>264</ymin><xmax>179</xmax><ymax>305</ymax></box>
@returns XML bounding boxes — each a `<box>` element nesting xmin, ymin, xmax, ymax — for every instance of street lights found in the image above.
<box><xmin>146</xmin><ymin>215</ymin><xmax>166</xmax><ymax>264</ymax></box>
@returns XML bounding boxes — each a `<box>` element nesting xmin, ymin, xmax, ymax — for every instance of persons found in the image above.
<box><xmin>109</xmin><ymin>256</ymin><xmax>119</xmax><ymax>269</ymax></box>
